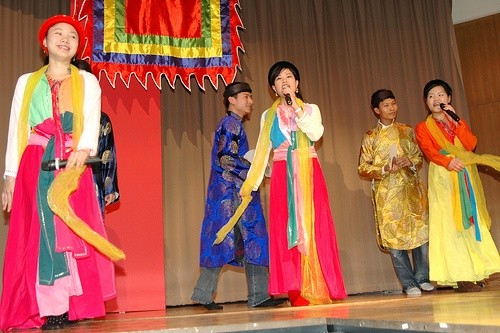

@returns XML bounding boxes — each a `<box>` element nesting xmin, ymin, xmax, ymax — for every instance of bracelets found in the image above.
<box><xmin>77</xmin><ymin>149</ymin><xmax>89</xmax><ymax>155</ymax></box>
<box><xmin>294</xmin><ymin>106</ymin><xmax>303</xmax><ymax>113</ymax></box>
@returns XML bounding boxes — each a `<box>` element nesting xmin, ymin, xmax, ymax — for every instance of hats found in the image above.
<box><xmin>38</xmin><ymin>13</ymin><xmax>86</xmax><ymax>57</ymax></box>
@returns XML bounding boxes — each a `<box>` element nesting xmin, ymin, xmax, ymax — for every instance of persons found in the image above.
<box><xmin>358</xmin><ymin>89</ymin><xmax>435</xmax><ymax>298</ymax></box>
<box><xmin>238</xmin><ymin>60</ymin><xmax>346</xmax><ymax>307</ymax></box>
<box><xmin>190</xmin><ymin>82</ymin><xmax>285</xmax><ymax>310</ymax></box>
<box><xmin>71</xmin><ymin>60</ymin><xmax>120</xmax><ymax>227</ymax></box>
<box><xmin>415</xmin><ymin>78</ymin><xmax>500</xmax><ymax>293</ymax></box>
<box><xmin>1</xmin><ymin>14</ymin><xmax>125</xmax><ymax>332</ymax></box>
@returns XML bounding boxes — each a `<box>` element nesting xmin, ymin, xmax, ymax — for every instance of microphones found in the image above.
<box><xmin>440</xmin><ymin>103</ymin><xmax>460</xmax><ymax>122</ymax></box>
<box><xmin>40</xmin><ymin>157</ymin><xmax>100</xmax><ymax>171</ymax></box>
<box><xmin>282</xmin><ymin>84</ymin><xmax>292</xmax><ymax>106</ymax></box>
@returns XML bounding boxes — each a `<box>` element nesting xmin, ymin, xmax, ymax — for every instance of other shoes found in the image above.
<box><xmin>456</xmin><ymin>280</ymin><xmax>487</xmax><ymax>291</ymax></box>
<box><xmin>40</xmin><ymin>313</ymin><xmax>70</xmax><ymax>330</ymax></box>
